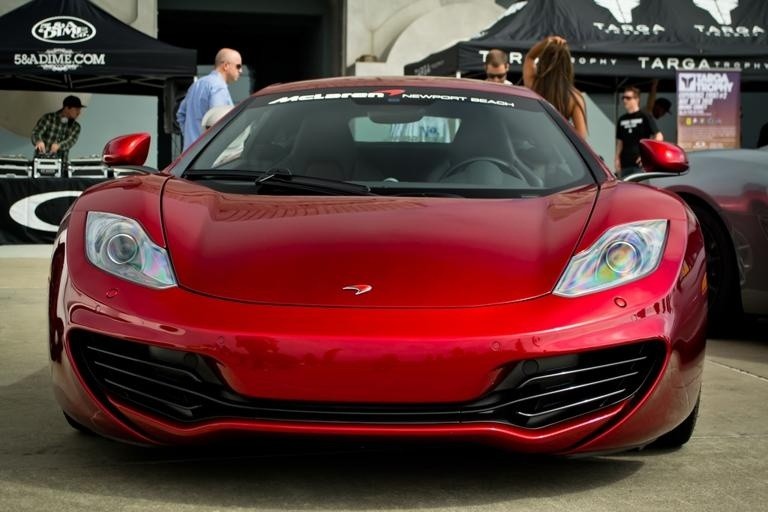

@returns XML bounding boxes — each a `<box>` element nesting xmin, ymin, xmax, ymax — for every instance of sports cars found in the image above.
<box><xmin>40</xmin><ymin>73</ymin><xmax>710</xmax><ymax>463</ymax></box>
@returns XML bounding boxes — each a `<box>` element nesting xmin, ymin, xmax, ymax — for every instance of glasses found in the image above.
<box><xmin>485</xmin><ymin>69</ymin><xmax>508</xmax><ymax>80</ymax></box>
<box><xmin>221</xmin><ymin>60</ymin><xmax>242</xmax><ymax>71</ymax></box>
<box><xmin>621</xmin><ymin>95</ymin><xmax>637</xmax><ymax>101</ymax></box>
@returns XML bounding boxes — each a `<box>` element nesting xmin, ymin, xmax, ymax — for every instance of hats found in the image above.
<box><xmin>63</xmin><ymin>95</ymin><xmax>88</xmax><ymax>108</ymax></box>
<box><xmin>654</xmin><ymin>97</ymin><xmax>673</xmax><ymax>116</ymax></box>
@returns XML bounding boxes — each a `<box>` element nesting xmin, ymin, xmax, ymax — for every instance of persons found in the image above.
<box><xmin>522</xmin><ymin>35</ymin><xmax>590</xmax><ymax>141</ymax></box>
<box><xmin>613</xmin><ymin>85</ymin><xmax>664</xmax><ymax>181</ymax></box>
<box><xmin>28</xmin><ymin>94</ymin><xmax>88</xmax><ymax>177</ymax></box>
<box><xmin>483</xmin><ymin>48</ymin><xmax>514</xmax><ymax>86</ymax></box>
<box><xmin>173</xmin><ymin>46</ymin><xmax>244</xmax><ymax>154</ymax></box>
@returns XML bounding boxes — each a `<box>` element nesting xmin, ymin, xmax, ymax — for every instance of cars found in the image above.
<box><xmin>617</xmin><ymin>126</ymin><xmax>766</xmax><ymax>341</ymax></box>
<box><xmin>709</xmin><ymin>188</ymin><xmax>768</xmax><ymax>289</ymax></box>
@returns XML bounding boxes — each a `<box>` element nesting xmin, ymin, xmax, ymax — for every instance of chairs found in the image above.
<box><xmin>424</xmin><ymin>109</ymin><xmax>542</xmax><ymax>189</ymax></box>
<box><xmin>269</xmin><ymin>108</ymin><xmax>378</xmax><ymax>182</ymax></box>
